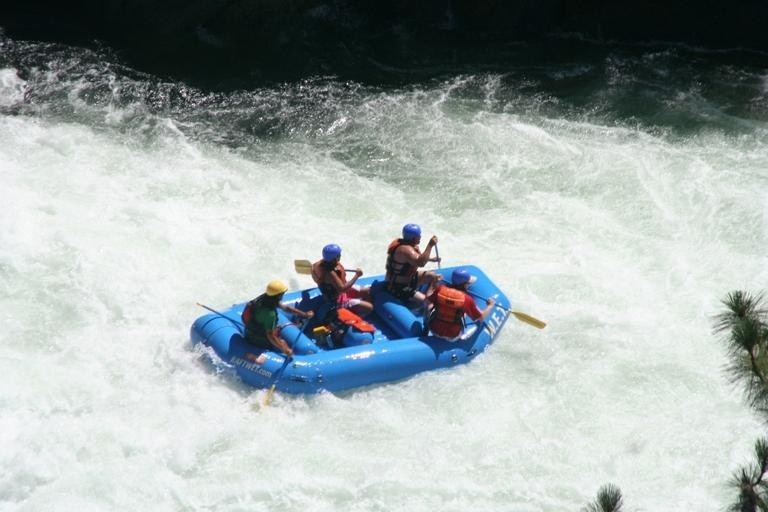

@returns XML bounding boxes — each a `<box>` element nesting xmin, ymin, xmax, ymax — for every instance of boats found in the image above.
<box><xmin>191</xmin><ymin>265</ymin><xmax>511</xmax><ymax>395</ymax></box>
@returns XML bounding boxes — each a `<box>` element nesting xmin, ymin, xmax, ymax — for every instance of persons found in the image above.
<box><xmin>425</xmin><ymin>268</ymin><xmax>496</xmax><ymax>342</ymax></box>
<box><xmin>384</xmin><ymin>224</ymin><xmax>439</xmax><ymax>314</ymax></box>
<box><xmin>240</xmin><ymin>279</ymin><xmax>315</xmax><ymax>357</ymax></box>
<box><xmin>311</xmin><ymin>244</ymin><xmax>376</xmax><ymax>320</ymax></box>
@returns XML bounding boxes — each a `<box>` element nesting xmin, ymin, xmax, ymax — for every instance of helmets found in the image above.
<box><xmin>403</xmin><ymin>224</ymin><xmax>420</xmax><ymax>241</ymax></box>
<box><xmin>323</xmin><ymin>244</ymin><xmax>341</xmax><ymax>262</ymax></box>
<box><xmin>266</xmin><ymin>281</ymin><xmax>288</xmax><ymax>297</ymax></box>
<box><xmin>453</xmin><ymin>269</ymin><xmax>478</xmax><ymax>285</ymax></box>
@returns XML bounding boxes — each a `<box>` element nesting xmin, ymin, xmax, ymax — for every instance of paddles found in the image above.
<box><xmin>440</xmin><ymin>278</ymin><xmax>546</xmax><ymax>329</ymax></box>
<box><xmin>261</xmin><ymin>317</ymin><xmax>311</xmax><ymax>406</ymax></box>
<box><xmin>294</xmin><ymin>260</ymin><xmax>357</xmax><ymax>274</ymax></box>
